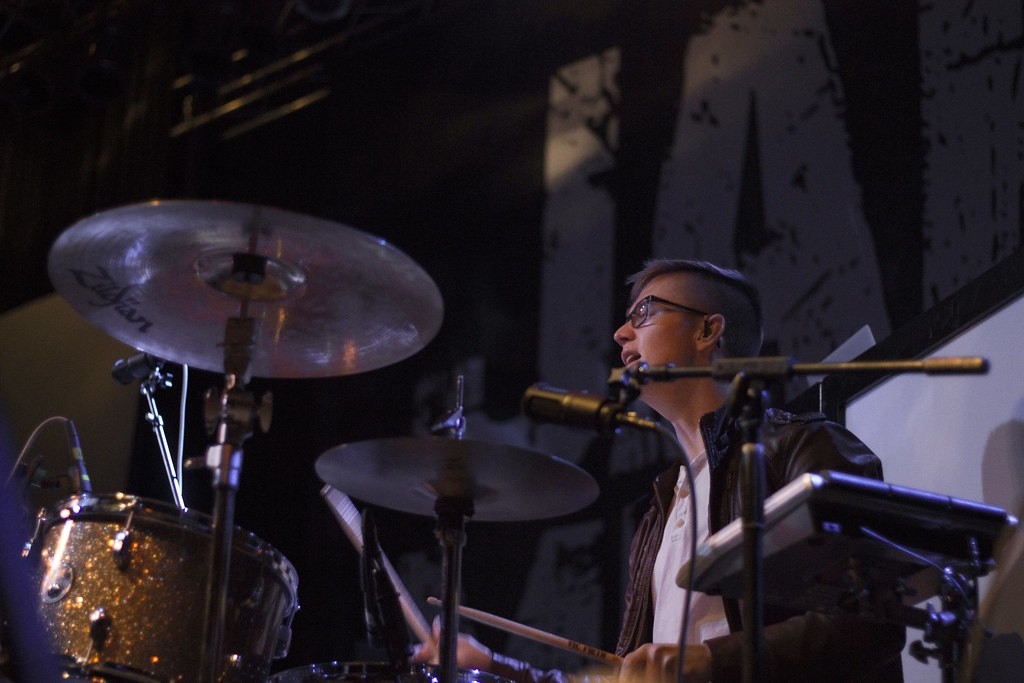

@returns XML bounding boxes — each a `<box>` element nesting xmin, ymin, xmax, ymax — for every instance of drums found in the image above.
<box><xmin>12</xmin><ymin>494</ymin><xmax>302</xmax><ymax>683</ymax></box>
<box><xmin>265</xmin><ymin>661</ymin><xmax>515</xmax><ymax>683</ymax></box>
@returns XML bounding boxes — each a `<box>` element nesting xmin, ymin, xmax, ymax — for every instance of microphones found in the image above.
<box><xmin>68</xmin><ymin>420</ymin><xmax>93</xmax><ymax>497</ymax></box>
<box><xmin>111</xmin><ymin>353</ymin><xmax>166</xmax><ymax>385</ymax></box>
<box><xmin>360</xmin><ymin>510</ymin><xmax>388</xmax><ymax>650</ymax></box>
<box><xmin>523</xmin><ymin>383</ymin><xmax>659</xmax><ymax>432</ymax></box>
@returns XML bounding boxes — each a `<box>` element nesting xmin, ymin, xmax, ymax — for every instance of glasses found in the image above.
<box><xmin>625</xmin><ymin>295</ymin><xmax>721</xmax><ymax>349</ymax></box>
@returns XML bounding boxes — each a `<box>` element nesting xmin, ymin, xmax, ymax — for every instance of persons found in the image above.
<box><xmin>412</xmin><ymin>258</ymin><xmax>907</xmax><ymax>683</ymax></box>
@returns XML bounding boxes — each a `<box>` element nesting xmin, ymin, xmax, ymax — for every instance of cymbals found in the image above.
<box><xmin>317</xmin><ymin>435</ymin><xmax>600</xmax><ymax>524</ymax></box>
<box><xmin>49</xmin><ymin>202</ymin><xmax>443</xmax><ymax>376</ymax></box>
